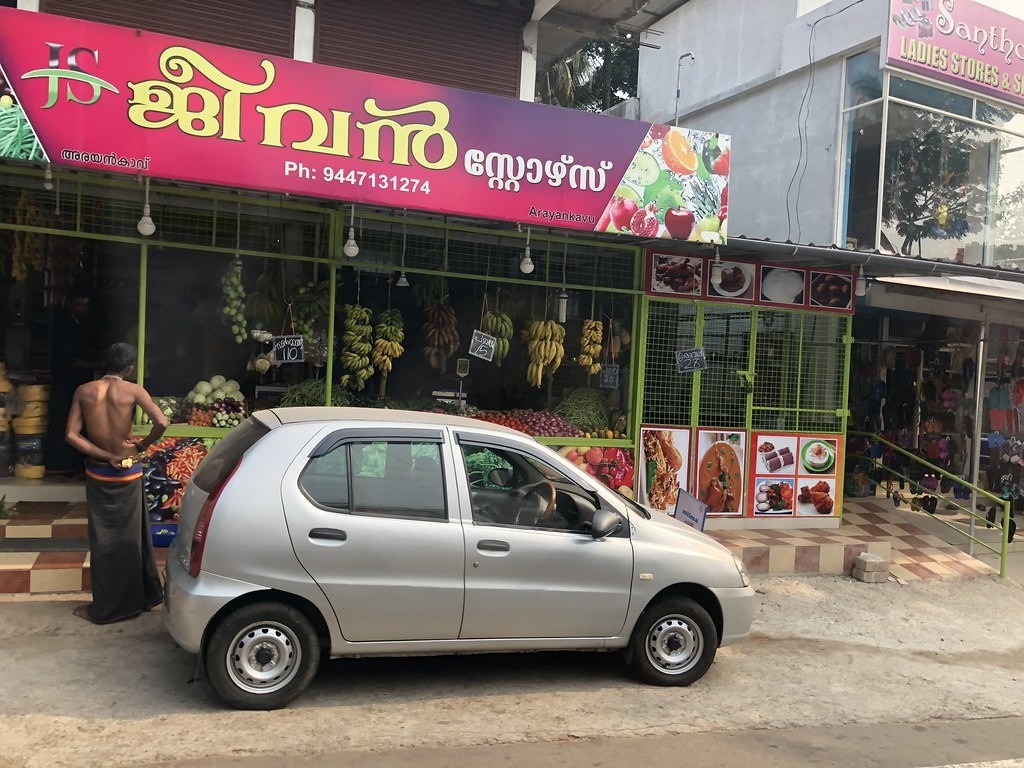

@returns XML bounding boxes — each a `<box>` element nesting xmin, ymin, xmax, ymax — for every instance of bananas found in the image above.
<box><xmin>340</xmin><ymin>303</ymin><xmax>603</xmax><ymax>391</ymax></box>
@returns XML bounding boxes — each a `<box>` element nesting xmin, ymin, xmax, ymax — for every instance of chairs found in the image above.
<box><xmin>304</xmin><ymin>453</ymin><xmax>496</xmax><ymax>524</ymax></box>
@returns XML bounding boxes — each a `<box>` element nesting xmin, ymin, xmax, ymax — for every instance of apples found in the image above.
<box><xmin>665</xmin><ymin>205</ymin><xmax>695</xmax><ymax>240</ymax></box>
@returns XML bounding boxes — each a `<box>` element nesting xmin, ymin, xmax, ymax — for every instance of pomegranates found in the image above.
<box><xmin>609</xmin><ymin>195</ymin><xmax>659</xmax><ymax>237</ymax></box>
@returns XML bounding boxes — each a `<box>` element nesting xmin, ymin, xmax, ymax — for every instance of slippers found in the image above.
<box><xmin>845</xmin><ymin>336</ymin><xmax>1024</xmax><ymax>543</ymax></box>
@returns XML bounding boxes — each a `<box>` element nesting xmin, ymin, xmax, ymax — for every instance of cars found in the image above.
<box><xmin>157</xmin><ymin>406</ymin><xmax>759</xmax><ymax>713</ymax></box>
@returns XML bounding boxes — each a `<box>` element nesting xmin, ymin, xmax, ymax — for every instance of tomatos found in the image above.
<box><xmin>475</xmin><ymin>412</ymin><xmax>533</xmax><ymax>436</ymax></box>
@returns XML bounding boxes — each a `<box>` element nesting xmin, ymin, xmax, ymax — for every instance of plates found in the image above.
<box><xmin>642</xmin><ymin>431</ymin><xmax>686</xmax><ymax>511</ymax></box>
<box><xmin>653</xmin><ymin>268</ymin><xmax>701</xmax><ymax>294</ymax></box>
<box><xmin>801</xmin><ymin>440</ymin><xmax>834</xmax><ymax>471</ymax></box>
<box><xmin>810</xmin><ymin>274</ymin><xmax>852</xmax><ymax>307</ymax></box>
<box><xmin>762</xmin><ymin>269</ymin><xmax>803</xmax><ymax>302</ymax></box>
<box><xmin>700</xmin><ymin>441</ymin><xmax>742</xmax><ymax>513</ymax></box>
<box><xmin>712</xmin><ymin>263</ymin><xmax>751</xmax><ymax>296</ymax></box>
<box><xmin>755</xmin><ymin>481</ymin><xmax>792</xmax><ymax>513</ymax></box>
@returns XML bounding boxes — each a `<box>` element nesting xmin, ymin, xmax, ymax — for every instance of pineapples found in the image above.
<box><xmin>611</xmin><ymin>322</ymin><xmax>630</xmax><ymax>354</ymax></box>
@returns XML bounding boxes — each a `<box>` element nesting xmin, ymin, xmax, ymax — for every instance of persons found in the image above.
<box><xmin>66</xmin><ymin>340</ymin><xmax>167</xmax><ymax>623</ymax></box>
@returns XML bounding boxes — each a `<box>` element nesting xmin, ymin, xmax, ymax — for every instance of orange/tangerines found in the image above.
<box><xmin>661</xmin><ymin>130</ymin><xmax>698</xmax><ymax>175</ymax></box>
